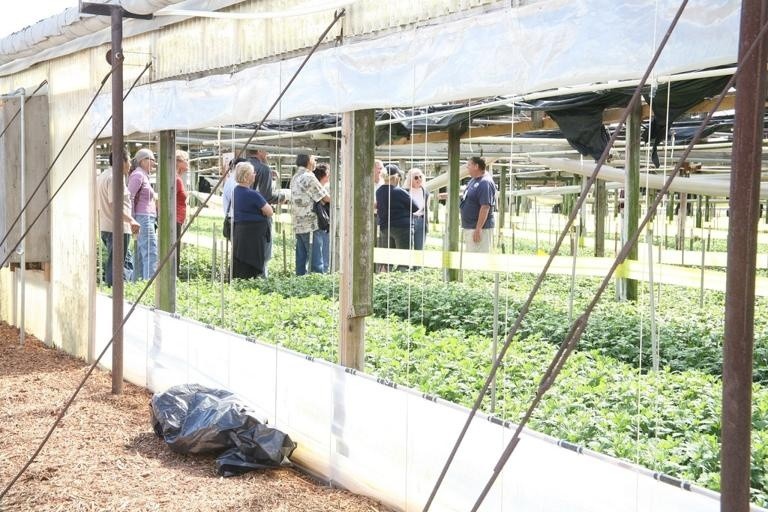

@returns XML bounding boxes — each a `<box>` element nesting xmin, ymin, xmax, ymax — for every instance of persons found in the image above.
<box><xmin>224</xmin><ymin>150</ymin><xmax>431</xmax><ymax>284</ymax></box>
<box><xmin>96</xmin><ymin>149</ymin><xmax>189</xmax><ymax>288</ymax></box>
<box><xmin>460</xmin><ymin>157</ymin><xmax>496</xmax><ymax>253</ymax></box>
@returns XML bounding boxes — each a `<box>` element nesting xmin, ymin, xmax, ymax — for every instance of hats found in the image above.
<box><xmin>385</xmin><ymin>164</ymin><xmax>402</xmax><ymax>176</ymax></box>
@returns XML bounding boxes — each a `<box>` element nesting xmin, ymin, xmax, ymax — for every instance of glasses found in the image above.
<box><xmin>415</xmin><ymin>175</ymin><xmax>423</xmax><ymax>181</ymax></box>
<box><xmin>144</xmin><ymin>156</ymin><xmax>155</xmax><ymax>162</ymax></box>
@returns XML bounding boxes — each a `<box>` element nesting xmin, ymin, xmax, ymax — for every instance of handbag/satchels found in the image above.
<box><xmin>223</xmin><ymin>216</ymin><xmax>231</xmax><ymax>240</ymax></box>
<box><xmin>314</xmin><ymin>201</ymin><xmax>330</xmax><ymax>233</ymax></box>
